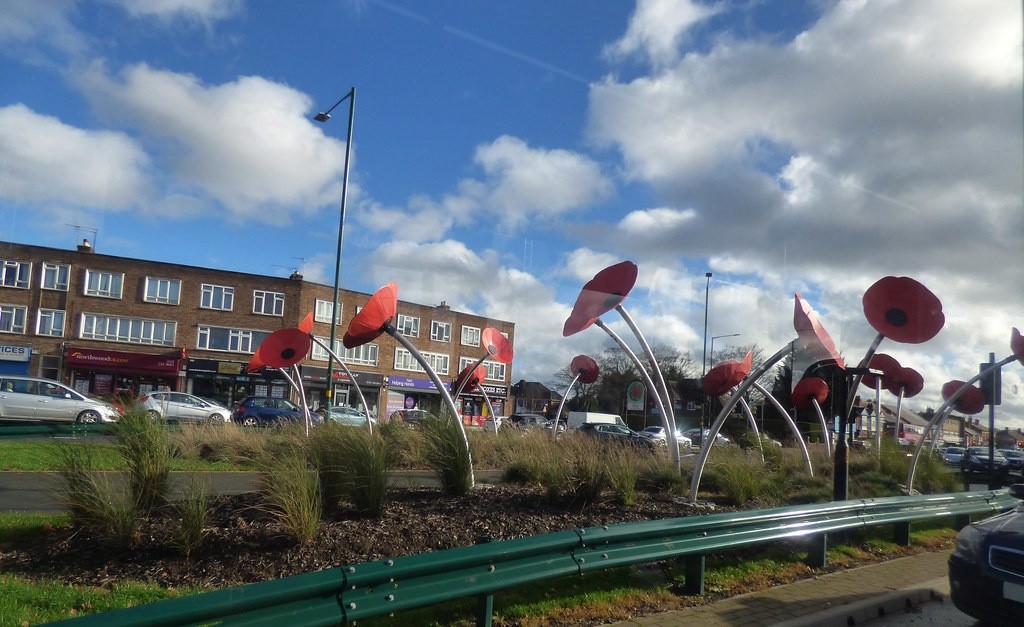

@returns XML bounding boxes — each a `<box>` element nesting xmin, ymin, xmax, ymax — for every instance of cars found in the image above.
<box><xmin>682</xmin><ymin>428</ymin><xmax>730</xmax><ymax>447</ymax></box>
<box><xmin>997</xmin><ymin>448</ymin><xmax>1024</xmax><ymax>470</ymax></box>
<box><xmin>849</xmin><ymin>439</ymin><xmax>871</xmax><ymax>448</ymax></box>
<box><xmin>546</xmin><ymin>419</ymin><xmax>566</xmax><ymax>431</ymax></box>
<box><xmin>739</xmin><ymin>431</ymin><xmax>783</xmax><ymax>448</ymax></box>
<box><xmin>898</xmin><ymin>437</ymin><xmax>914</xmax><ymax>446</ymax></box>
<box><xmin>961</xmin><ymin>448</ymin><xmax>1009</xmax><ymax>475</ymax></box>
<box><xmin>316</xmin><ymin>406</ymin><xmax>376</xmax><ymax>429</ymax></box>
<box><xmin>0</xmin><ymin>374</ymin><xmax>120</xmax><ymax>426</ymax></box>
<box><xmin>233</xmin><ymin>395</ymin><xmax>320</xmax><ymax>428</ymax></box>
<box><xmin>935</xmin><ymin>447</ymin><xmax>965</xmax><ymax>467</ymax></box>
<box><xmin>637</xmin><ymin>426</ymin><xmax>692</xmax><ymax>449</ymax></box>
<box><xmin>390</xmin><ymin>410</ymin><xmax>438</xmax><ymax>428</ymax></box>
<box><xmin>138</xmin><ymin>391</ymin><xmax>232</xmax><ymax>425</ymax></box>
<box><xmin>947</xmin><ymin>484</ymin><xmax>1024</xmax><ymax>627</ymax></box>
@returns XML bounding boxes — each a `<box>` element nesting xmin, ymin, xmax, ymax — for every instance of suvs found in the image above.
<box><xmin>482</xmin><ymin>416</ymin><xmax>509</xmax><ymax>433</ymax></box>
<box><xmin>575</xmin><ymin>422</ymin><xmax>658</xmax><ymax>453</ymax></box>
<box><xmin>497</xmin><ymin>414</ymin><xmax>549</xmax><ymax>436</ymax></box>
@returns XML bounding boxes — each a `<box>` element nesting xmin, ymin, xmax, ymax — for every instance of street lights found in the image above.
<box><xmin>810</xmin><ymin>364</ymin><xmax>884</xmax><ymax>501</ymax></box>
<box><xmin>313</xmin><ymin>86</ymin><xmax>357</xmax><ymax>431</ymax></box>
<box><xmin>708</xmin><ymin>334</ymin><xmax>740</xmax><ymax>426</ymax></box>
<box><xmin>703</xmin><ymin>273</ymin><xmax>713</xmax><ymax>378</ymax></box>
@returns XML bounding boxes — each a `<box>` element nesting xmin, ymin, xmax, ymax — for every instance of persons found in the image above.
<box><xmin>38</xmin><ymin>382</ymin><xmax>64</xmax><ymax>395</ymax></box>
<box><xmin>962</xmin><ymin>449</ymin><xmax>971</xmax><ymax>491</ymax></box>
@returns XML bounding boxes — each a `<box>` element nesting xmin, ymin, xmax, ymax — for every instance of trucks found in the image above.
<box><xmin>566</xmin><ymin>412</ymin><xmax>626</xmax><ymax>429</ymax></box>
